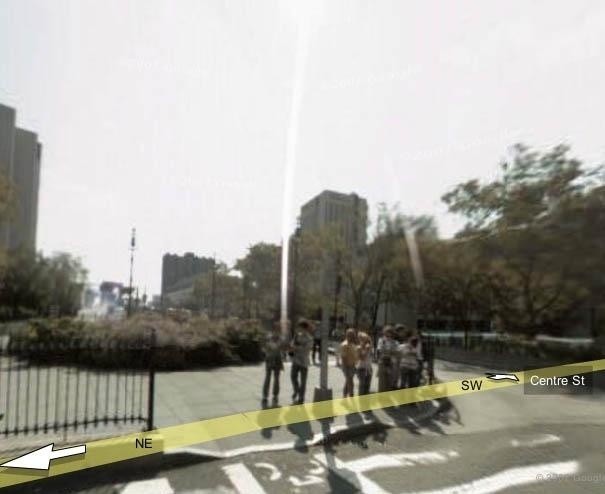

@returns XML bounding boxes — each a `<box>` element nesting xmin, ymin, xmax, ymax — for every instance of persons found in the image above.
<box><xmin>336</xmin><ymin>321</ymin><xmax>437</xmax><ymax>399</ymax></box>
<box><xmin>258</xmin><ymin>321</ymin><xmax>286</xmax><ymax>401</ymax></box>
<box><xmin>287</xmin><ymin>318</ymin><xmax>313</xmax><ymax>404</ymax></box>
<box><xmin>312</xmin><ymin>323</ymin><xmax>322</xmax><ymax>363</ymax></box>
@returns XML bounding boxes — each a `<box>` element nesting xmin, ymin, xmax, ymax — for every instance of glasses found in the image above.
<box><xmin>385</xmin><ymin>334</ymin><xmax>392</xmax><ymax>337</ymax></box>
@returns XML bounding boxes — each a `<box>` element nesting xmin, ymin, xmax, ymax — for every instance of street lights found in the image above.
<box><xmin>290</xmin><ymin>214</ymin><xmax>304</xmax><ymax>338</ymax></box>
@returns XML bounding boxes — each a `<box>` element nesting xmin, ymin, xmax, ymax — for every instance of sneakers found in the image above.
<box><xmin>261</xmin><ymin>395</ymin><xmax>279</xmax><ymax>405</ymax></box>
<box><xmin>312</xmin><ymin>360</ymin><xmax>315</xmax><ymax>364</ymax></box>
<box><xmin>292</xmin><ymin>389</ymin><xmax>304</xmax><ymax>404</ymax></box>
